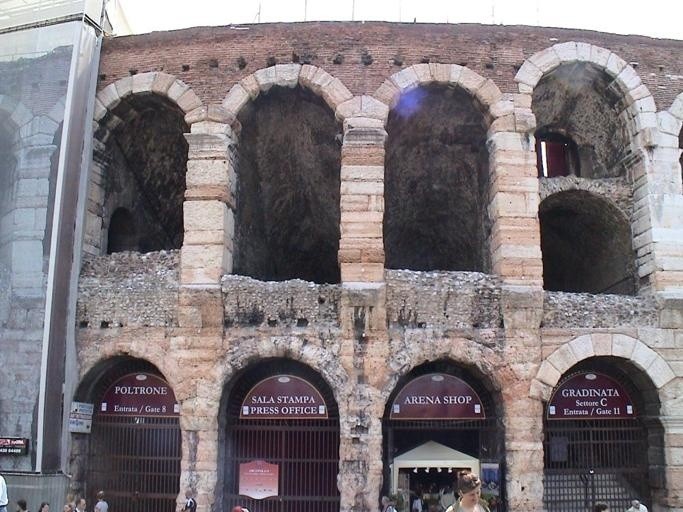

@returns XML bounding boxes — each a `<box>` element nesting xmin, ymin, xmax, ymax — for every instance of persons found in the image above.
<box><xmin>0</xmin><ymin>473</ymin><xmax>110</xmax><ymax>512</ymax></box>
<box><xmin>182</xmin><ymin>489</ymin><xmax>198</xmax><ymax>512</ymax></box>
<box><xmin>593</xmin><ymin>502</ymin><xmax>609</xmax><ymax>512</ymax></box>
<box><xmin>624</xmin><ymin>498</ymin><xmax>648</xmax><ymax>512</ymax></box>
<box><xmin>444</xmin><ymin>470</ymin><xmax>492</xmax><ymax>511</ymax></box>
<box><xmin>380</xmin><ymin>483</ymin><xmax>456</xmax><ymax>512</ymax></box>
